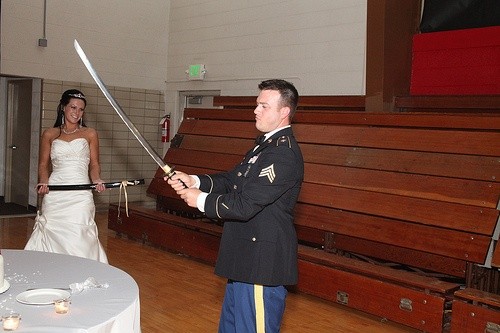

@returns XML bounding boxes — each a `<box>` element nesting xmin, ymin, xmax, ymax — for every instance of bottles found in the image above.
<box><xmin>0</xmin><ymin>249</ymin><xmax>4</xmax><ymax>288</ymax></box>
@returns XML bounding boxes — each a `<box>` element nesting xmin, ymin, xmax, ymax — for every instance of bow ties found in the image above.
<box><xmin>255</xmin><ymin>135</ymin><xmax>266</xmax><ymax>145</ymax></box>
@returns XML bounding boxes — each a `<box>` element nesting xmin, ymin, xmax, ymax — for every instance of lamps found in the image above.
<box><xmin>185</xmin><ymin>64</ymin><xmax>208</xmax><ymax>81</ymax></box>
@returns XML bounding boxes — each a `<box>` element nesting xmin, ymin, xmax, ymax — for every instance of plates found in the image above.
<box><xmin>0</xmin><ymin>279</ymin><xmax>11</xmax><ymax>294</ymax></box>
<box><xmin>17</xmin><ymin>287</ymin><xmax>70</xmax><ymax>306</ymax></box>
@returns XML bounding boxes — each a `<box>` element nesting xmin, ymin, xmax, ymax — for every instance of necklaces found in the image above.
<box><xmin>61</xmin><ymin>125</ymin><xmax>79</xmax><ymax>134</ymax></box>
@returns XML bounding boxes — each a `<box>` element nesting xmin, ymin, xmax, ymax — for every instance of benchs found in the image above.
<box><xmin>450</xmin><ymin>287</ymin><xmax>500</xmax><ymax>333</ymax></box>
<box><xmin>106</xmin><ymin>202</ymin><xmax>460</xmax><ymax>333</ymax></box>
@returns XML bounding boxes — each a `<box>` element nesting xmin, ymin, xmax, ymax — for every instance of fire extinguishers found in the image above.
<box><xmin>160</xmin><ymin>114</ymin><xmax>170</xmax><ymax>142</ymax></box>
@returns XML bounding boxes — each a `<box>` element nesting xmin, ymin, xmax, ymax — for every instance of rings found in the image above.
<box><xmin>98</xmin><ymin>191</ymin><xmax>101</xmax><ymax>193</ymax></box>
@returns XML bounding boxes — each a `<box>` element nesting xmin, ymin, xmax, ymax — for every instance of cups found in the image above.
<box><xmin>55</xmin><ymin>300</ymin><xmax>67</xmax><ymax>313</ymax></box>
<box><xmin>3</xmin><ymin>314</ymin><xmax>18</xmax><ymax>329</ymax></box>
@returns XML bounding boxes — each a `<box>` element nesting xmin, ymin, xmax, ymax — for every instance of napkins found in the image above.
<box><xmin>69</xmin><ymin>277</ymin><xmax>110</xmax><ymax>295</ymax></box>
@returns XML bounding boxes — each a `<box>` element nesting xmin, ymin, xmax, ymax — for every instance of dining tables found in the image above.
<box><xmin>0</xmin><ymin>249</ymin><xmax>140</xmax><ymax>333</ymax></box>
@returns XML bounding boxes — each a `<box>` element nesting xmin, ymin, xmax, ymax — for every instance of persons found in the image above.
<box><xmin>24</xmin><ymin>88</ymin><xmax>109</xmax><ymax>265</ymax></box>
<box><xmin>163</xmin><ymin>79</ymin><xmax>304</xmax><ymax>333</ymax></box>
